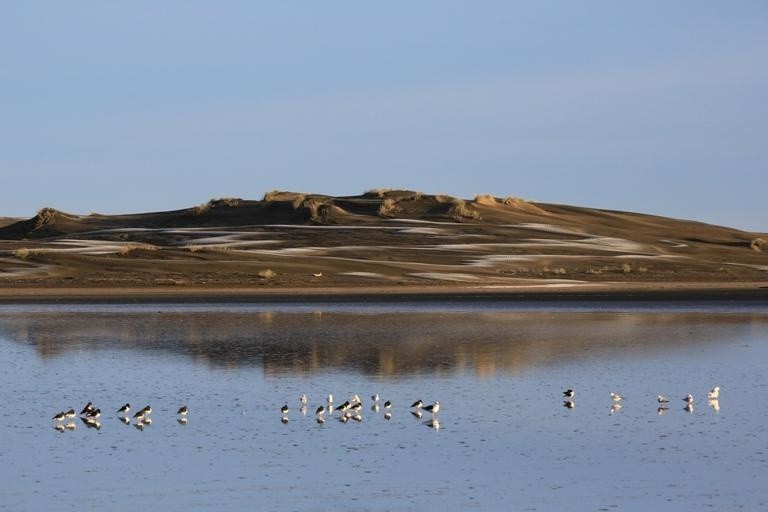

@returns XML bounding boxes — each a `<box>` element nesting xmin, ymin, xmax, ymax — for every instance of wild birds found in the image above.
<box><xmin>611</xmin><ymin>392</ymin><xmax>625</xmax><ymax>402</ymax></box>
<box><xmin>176</xmin><ymin>416</ymin><xmax>189</xmax><ymax>426</ymax></box>
<box><xmin>177</xmin><ymin>405</ymin><xmax>189</xmax><ymax>417</ymax></box>
<box><xmin>53</xmin><ymin>416</ymin><xmax>152</xmax><ymax>433</ymax></box>
<box><xmin>611</xmin><ymin>404</ymin><xmax>621</xmax><ymax>414</ymax></box>
<box><xmin>656</xmin><ymin>386</ymin><xmax>720</xmax><ymax>405</ymax></box>
<box><xmin>564</xmin><ymin>389</ymin><xmax>575</xmax><ymax>399</ymax></box>
<box><xmin>280</xmin><ymin>410</ymin><xmax>440</xmax><ymax>432</ymax></box>
<box><xmin>563</xmin><ymin>400</ymin><xmax>574</xmax><ymax>410</ymax></box>
<box><xmin>50</xmin><ymin>402</ymin><xmax>152</xmax><ymax>424</ymax></box>
<box><xmin>281</xmin><ymin>393</ymin><xmax>440</xmax><ymax>416</ymax></box>
<box><xmin>656</xmin><ymin>399</ymin><xmax>720</xmax><ymax>415</ymax></box>
